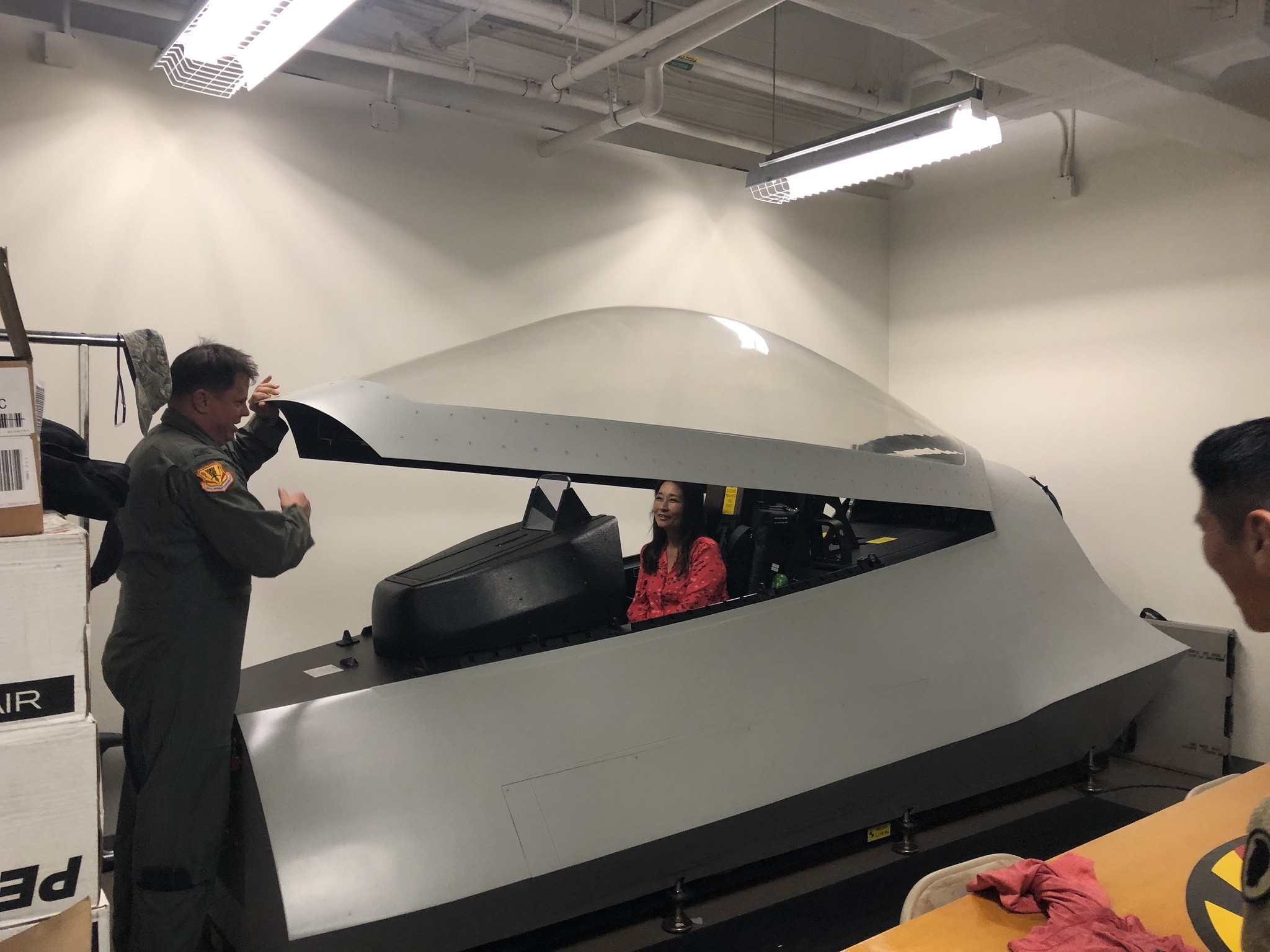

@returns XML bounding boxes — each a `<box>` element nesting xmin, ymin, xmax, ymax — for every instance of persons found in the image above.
<box><xmin>626</xmin><ymin>482</ymin><xmax>728</xmax><ymax>623</ymax></box>
<box><xmin>1193</xmin><ymin>416</ymin><xmax>1270</xmax><ymax>632</ymax></box>
<box><xmin>100</xmin><ymin>344</ymin><xmax>315</xmax><ymax>952</ymax></box>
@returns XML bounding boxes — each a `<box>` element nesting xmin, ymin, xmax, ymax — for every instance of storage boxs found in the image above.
<box><xmin>1</xmin><ymin>355</ymin><xmax>110</xmax><ymax>952</ymax></box>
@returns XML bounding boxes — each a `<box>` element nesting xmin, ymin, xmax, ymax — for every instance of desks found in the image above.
<box><xmin>840</xmin><ymin>761</ymin><xmax>1270</xmax><ymax>952</ymax></box>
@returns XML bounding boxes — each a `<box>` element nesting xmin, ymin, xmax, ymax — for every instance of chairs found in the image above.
<box><xmin>698</xmin><ymin>483</ymin><xmax>759</xmax><ymax>602</ymax></box>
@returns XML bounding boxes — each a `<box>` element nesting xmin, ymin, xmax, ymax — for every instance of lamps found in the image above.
<box><xmin>148</xmin><ymin>0</ymin><xmax>356</xmax><ymax>99</ymax></box>
<box><xmin>744</xmin><ymin>5</ymin><xmax>1002</xmax><ymax>205</ymax></box>
